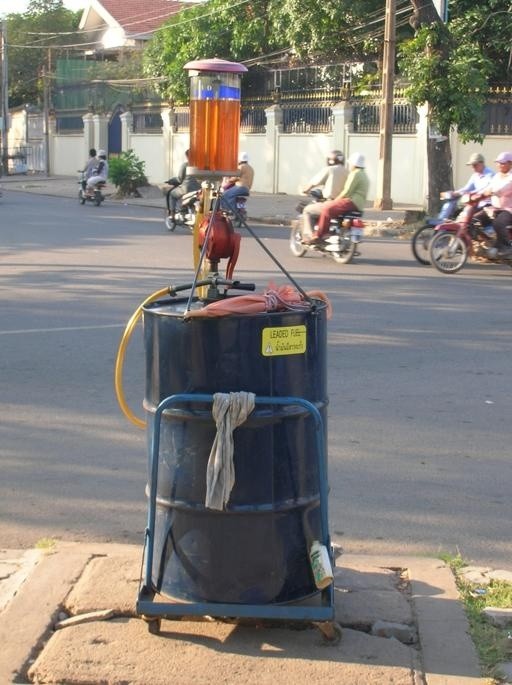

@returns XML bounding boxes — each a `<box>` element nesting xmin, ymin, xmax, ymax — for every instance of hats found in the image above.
<box><xmin>97</xmin><ymin>149</ymin><xmax>105</xmax><ymax>157</ymax></box>
<box><xmin>467</xmin><ymin>154</ymin><xmax>485</xmax><ymax>166</ymax></box>
<box><xmin>494</xmin><ymin>152</ymin><xmax>512</xmax><ymax>164</ymax></box>
<box><xmin>239</xmin><ymin>152</ymin><xmax>248</xmax><ymax>162</ymax></box>
<box><xmin>347</xmin><ymin>155</ymin><xmax>366</xmax><ymax>168</ymax></box>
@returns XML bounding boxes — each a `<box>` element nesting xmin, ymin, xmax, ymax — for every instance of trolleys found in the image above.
<box><xmin>136</xmin><ymin>393</ymin><xmax>343</xmax><ymax>646</ymax></box>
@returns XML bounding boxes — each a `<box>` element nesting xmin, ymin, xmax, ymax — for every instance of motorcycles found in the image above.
<box><xmin>164</xmin><ymin>177</ymin><xmax>221</xmax><ymax>230</ymax></box>
<box><xmin>411</xmin><ymin>191</ymin><xmax>512</xmax><ymax>265</ymax></box>
<box><xmin>430</xmin><ymin>193</ymin><xmax>512</xmax><ymax>274</ymax></box>
<box><xmin>77</xmin><ymin>171</ymin><xmax>106</xmax><ymax>206</ymax></box>
<box><xmin>290</xmin><ymin>190</ymin><xmax>363</xmax><ymax>265</ymax></box>
<box><xmin>221</xmin><ymin>186</ymin><xmax>249</xmax><ymax>228</ymax></box>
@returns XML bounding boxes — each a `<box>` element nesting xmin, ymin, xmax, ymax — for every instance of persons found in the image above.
<box><xmin>465</xmin><ymin>152</ymin><xmax>512</xmax><ymax>258</ymax></box>
<box><xmin>310</xmin><ymin>153</ymin><xmax>371</xmax><ymax>241</ymax></box>
<box><xmin>164</xmin><ymin>145</ymin><xmax>202</xmax><ymax>222</ymax></box>
<box><xmin>80</xmin><ymin>149</ymin><xmax>97</xmax><ymax>199</ymax></box>
<box><xmin>302</xmin><ymin>151</ymin><xmax>350</xmax><ymax>244</ymax></box>
<box><xmin>440</xmin><ymin>152</ymin><xmax>495</xmax><ymax>238</ymax></box>
<box><xmin>85</xmin><ymin>149</ymin><xmax>110</xmax><ymax>202</ymax></box>
<box><xmin>220</xmin><ymin>152</ymin><xmax>255</xmax><ymax>217</ymax></box>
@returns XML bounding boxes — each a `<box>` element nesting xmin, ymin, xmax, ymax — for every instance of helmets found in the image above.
<box><xmin>327</xmin><ymin>151</ymin><xmax>345</xmax><ymax>165</ymax></box>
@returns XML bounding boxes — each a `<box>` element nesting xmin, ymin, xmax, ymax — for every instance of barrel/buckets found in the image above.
<box><xmin>141</xmin><ymin>293</ymin><xmax>331</xmax><ymax>604</ymax></box>
